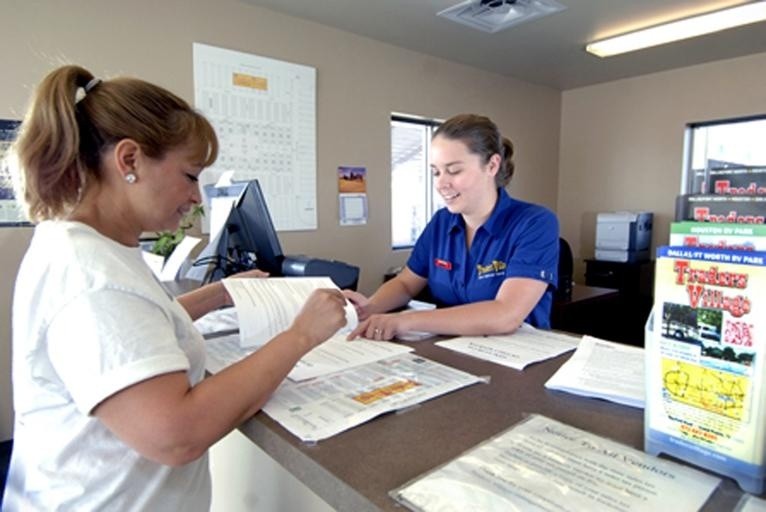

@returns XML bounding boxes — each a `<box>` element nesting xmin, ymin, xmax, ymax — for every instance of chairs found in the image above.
<box><xmin>551</xmin><ymin>237</ymin><xmax>573</xmax><ymax>332</ymax></box>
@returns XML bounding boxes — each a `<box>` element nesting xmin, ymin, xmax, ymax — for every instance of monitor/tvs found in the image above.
<box><xmin>204</xmin><ymin>179</ymin><xmax>283</xmax><ymax>267</ymax></box>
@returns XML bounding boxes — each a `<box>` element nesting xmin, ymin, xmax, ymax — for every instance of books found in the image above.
<box><xmin>544</xmin><ymin>334</ymin><xmax>648</xmax><ymax>411</ymax></box>
<box><xmin>649</xmin><ymin>157</ymin><xmax>766</xmax><ymax>466</ymax></box>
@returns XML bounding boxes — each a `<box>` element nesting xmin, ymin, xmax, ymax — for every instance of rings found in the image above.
<box><xmin>375</xmin><ymin>329</ymin><xmax>381</xmax><ymax>333</ymax></box>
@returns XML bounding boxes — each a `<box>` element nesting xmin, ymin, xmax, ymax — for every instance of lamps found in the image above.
<box><xmin>583</xmin><ymin>0</ymin><xmax>766</xmax><ymax>59</ymax></box>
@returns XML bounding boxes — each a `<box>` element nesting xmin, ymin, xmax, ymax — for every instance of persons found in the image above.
<box><xmin>341</xmin><ymin>115</ymin><xmax>559</xmax><ymax>344</ymax></box>
<box><xmin>0</xmin><ymin>63</ymin><xmax>350</xmax><ymax>512</ymax></box>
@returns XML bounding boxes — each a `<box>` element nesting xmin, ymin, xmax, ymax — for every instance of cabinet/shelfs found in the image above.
<box><xmin>584</xmin><ymin>259</ymin><xmax>655</xmax><ymax>345</ymax></box>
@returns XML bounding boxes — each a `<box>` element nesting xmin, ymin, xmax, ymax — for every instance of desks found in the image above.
<box><xmin>557</xmin><ymin>283</ymin><xmax>619</xmax><ymax>307</ymax></box>
